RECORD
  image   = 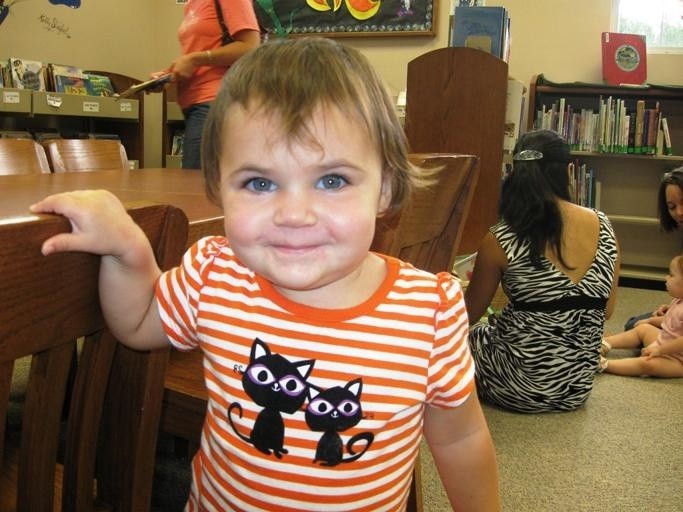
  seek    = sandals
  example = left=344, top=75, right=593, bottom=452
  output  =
left=597, top=339, right=612, bottom=374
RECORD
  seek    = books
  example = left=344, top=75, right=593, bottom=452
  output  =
left=535, top=95, right=673, bottom=156
left=601, top=31, right=647, bottom=87
left=113, top=72, right=175, bottom=103
left=0, top=55, right=115, bottom=96
left=447, top=5, right=510, bottom=64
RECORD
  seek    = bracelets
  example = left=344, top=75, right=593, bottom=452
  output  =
left=205, top=49, right=214, bottom=65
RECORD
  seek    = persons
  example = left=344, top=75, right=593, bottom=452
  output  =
left=30, top=34, right=506, bottom=512
left=146, top=1, right=264, bottom=169
left=657, top=166, right=683, bottom=232
left=463, top=130, right=623, bottom=416
left=597, top=253, right=683, bottom=380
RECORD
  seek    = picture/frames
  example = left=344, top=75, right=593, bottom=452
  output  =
left=252, top=0, right=440, bottom=39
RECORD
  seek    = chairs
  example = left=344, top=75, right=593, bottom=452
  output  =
left=0, top=135, right=482, bottom=512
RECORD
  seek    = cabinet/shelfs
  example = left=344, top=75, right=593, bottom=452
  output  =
left=1, top=69, right=185, bottom=170
left=404, top=47, right=526, bottom=254
left=520, top=75, right=683, bottom=283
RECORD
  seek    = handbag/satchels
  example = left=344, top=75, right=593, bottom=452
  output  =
left=223, top=32, right=273, bottom=69
